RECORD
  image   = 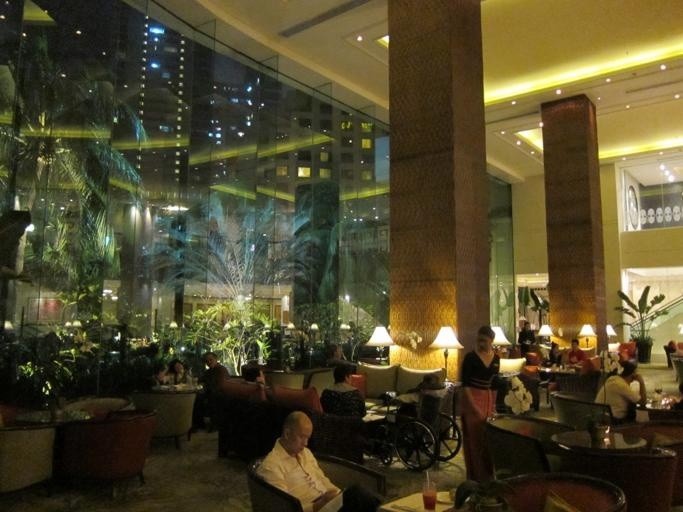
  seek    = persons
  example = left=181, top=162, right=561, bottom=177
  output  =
left=241, top=362, right=266, bottom=385
left=594, top=360, right=647, bottom=424
left=518, top=321, right=536, bottom=356
left=568, top=339, right=588, bottom=365
left=256, top=410, right=389, bottom=511
left=538, top=341, right=563, bottom=385
left=157, top=358, right=193, bottom=384
left=461, top=325, right=500, bottom=478
left=396, top=374, right=446, bottom=419
left=319, top=364, right=367, bottom=416
left=199, top=352, right=229, bottom=389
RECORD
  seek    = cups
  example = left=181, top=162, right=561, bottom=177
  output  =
left=655, top=383, right=663, bottom=394
left=422, top=481, right=438, bottom=510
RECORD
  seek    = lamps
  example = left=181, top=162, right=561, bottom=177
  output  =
left=432, top=326, right=464, bottom=369
left=607, top=325, right=617, bottom=342
left=490, top=324, right=511, bottom=352
left=366, top=326, right=393, bottom=364
left=539, top=324, right=552, bottom=344
left=579, top=323, right=596, bottom=347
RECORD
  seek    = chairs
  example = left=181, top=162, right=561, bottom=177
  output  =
left=0, top=340, right=683, bottom=512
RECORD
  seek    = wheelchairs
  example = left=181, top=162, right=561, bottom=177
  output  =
left=373, top=384, right=462, bottom=471
